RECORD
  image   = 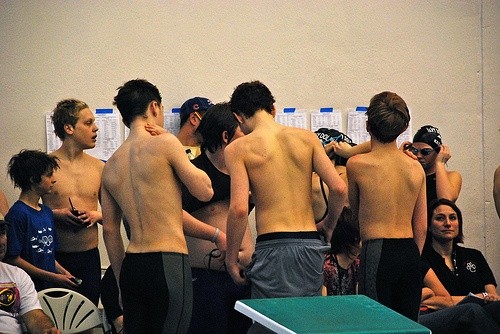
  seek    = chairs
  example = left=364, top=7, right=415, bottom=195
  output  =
left=37, top=287, right=104, bottom=334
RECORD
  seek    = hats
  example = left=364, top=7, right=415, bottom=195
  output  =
left=413, top=126, right=442, bottom=153
left=0, top=214, right=10, bottom=225
left=180, top=98, right=215, bottom=118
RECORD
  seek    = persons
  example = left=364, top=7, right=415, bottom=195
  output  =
left=312, top=128, right=372, bottom=296
left=400, top=125, right=500, bottom=334
left=346, top=91, right=427, bottom=322
left=223, top=81, right=347, bottom=334
left=100, top=215, right=131, bottom=334
left=0, top=190, right=62, bottom=334
left=182, top=103, right=256, bottom=334
left=176, top=97, right=214, bottom=160
left=101, top=80, right=215, bottom=334
left=40, top=99, right=105, bottom=334
left=493, top=166, right=500, bottom=220
left=1, top=149, right=78, bottom=328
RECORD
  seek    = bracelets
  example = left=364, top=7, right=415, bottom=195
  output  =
left=482, top=291, right=488, bottom=298
left=211, top=228, right=218, bottom=243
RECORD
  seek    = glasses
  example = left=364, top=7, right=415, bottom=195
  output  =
left=203, top=249, right=221, bottom=265
left=410, top=147, right=433, bottom=156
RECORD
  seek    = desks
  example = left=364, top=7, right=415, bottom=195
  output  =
left=234, top=295, right=432, bottom=334
